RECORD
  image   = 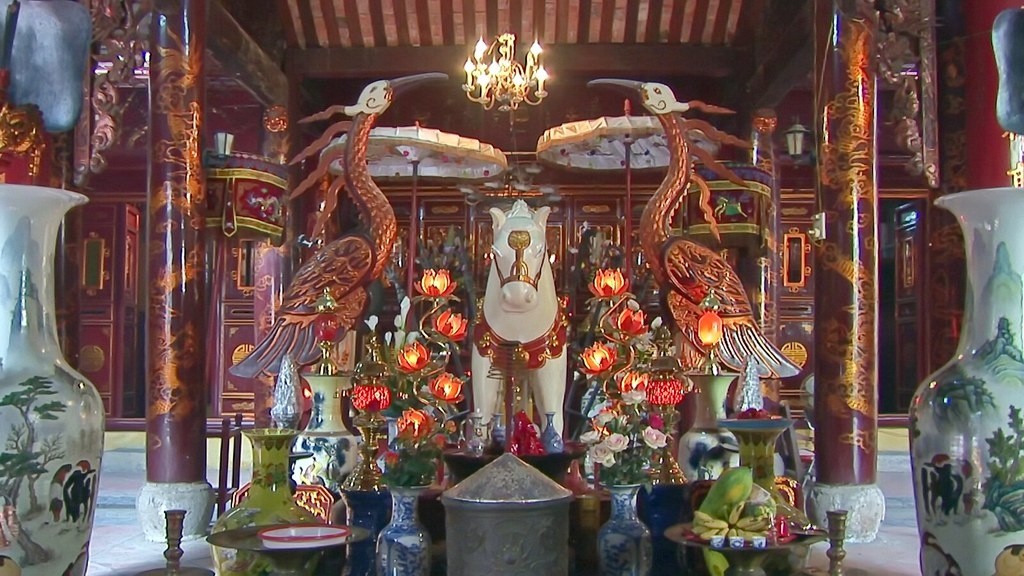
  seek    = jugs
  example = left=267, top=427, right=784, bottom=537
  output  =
left=457, top=408, right=491, bottom=458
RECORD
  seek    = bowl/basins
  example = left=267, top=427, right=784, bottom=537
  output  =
left=257, top=524, right=353, bottom=547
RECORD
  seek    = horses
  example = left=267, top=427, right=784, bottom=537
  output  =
left=471, top=200, right=567, bottom=439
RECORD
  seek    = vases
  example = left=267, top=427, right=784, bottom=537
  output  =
left=210, top=374, right=801, bottom=576
left=910, top=185, right=1024, bottom=576
left=0, top=183, right=106, bottom=576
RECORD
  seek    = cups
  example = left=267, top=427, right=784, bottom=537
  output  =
left=710, top=536, right=724, bottom=548
left=729, top=536, right=744, bottom=549
left=752, top=536, right=766, bottom=548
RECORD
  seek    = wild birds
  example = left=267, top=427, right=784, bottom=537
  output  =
left=589, top=78, right=803, bottom=379
left=227, top=73, right=449, bottom=379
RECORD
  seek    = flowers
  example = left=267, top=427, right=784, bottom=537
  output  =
left=583, top=267, right=651, bottom=401
left=397, top=265, right=469, bottom=434
left=364, top=294, right=424, bottom=413
left=630, top=298, right=675, bottom=359
left=579, top=384, right=670, bottom=490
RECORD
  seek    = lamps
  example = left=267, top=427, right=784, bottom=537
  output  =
left=203, top=129, right=235, bottom=166
left=780, top=113, right=813, bottom=169
left=462, top=29, right=549, bottom=114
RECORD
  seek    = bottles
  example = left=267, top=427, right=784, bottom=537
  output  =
left=492, top=414, right=507, bottom=453
left=909, top=188, right=1024, bottom=576
left=540, top=412, right=564, bottom=454
